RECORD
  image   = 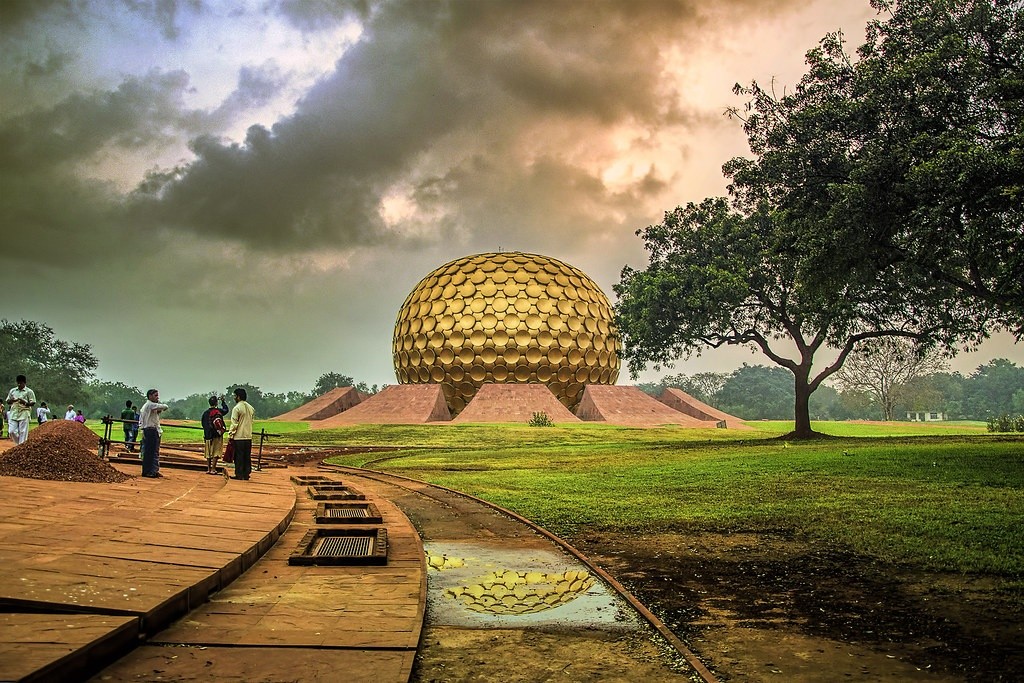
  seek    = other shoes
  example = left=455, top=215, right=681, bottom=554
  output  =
left=157, top=473, right=163, bottom=477
left=231, top=476, right=237, bottom=479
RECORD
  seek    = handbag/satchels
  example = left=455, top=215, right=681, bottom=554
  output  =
left=223, top=438, right=234, bottom=464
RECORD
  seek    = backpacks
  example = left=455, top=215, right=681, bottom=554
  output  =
left=209, top=407, right=226, bottom=436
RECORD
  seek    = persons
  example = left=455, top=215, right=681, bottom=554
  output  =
left=76, top=410, right=86, bottom=423
left=6, top=406, right=11, bottom=438
left=0, top=398, right=5, bottom=437
left=64, top=405, right=77, bottom=421
left=130, top=406, right=140, bottom=453
left=202, top=395, right=229, bottom=475
left=139, top=389, right=168, bottom=477
left=6, top=375, right=37, bottom=445
left=121, top=400, right=135, bottom=454
left=36, top=402, right=50, bottom=425
left=227, top=388, right=256, bottom=480
